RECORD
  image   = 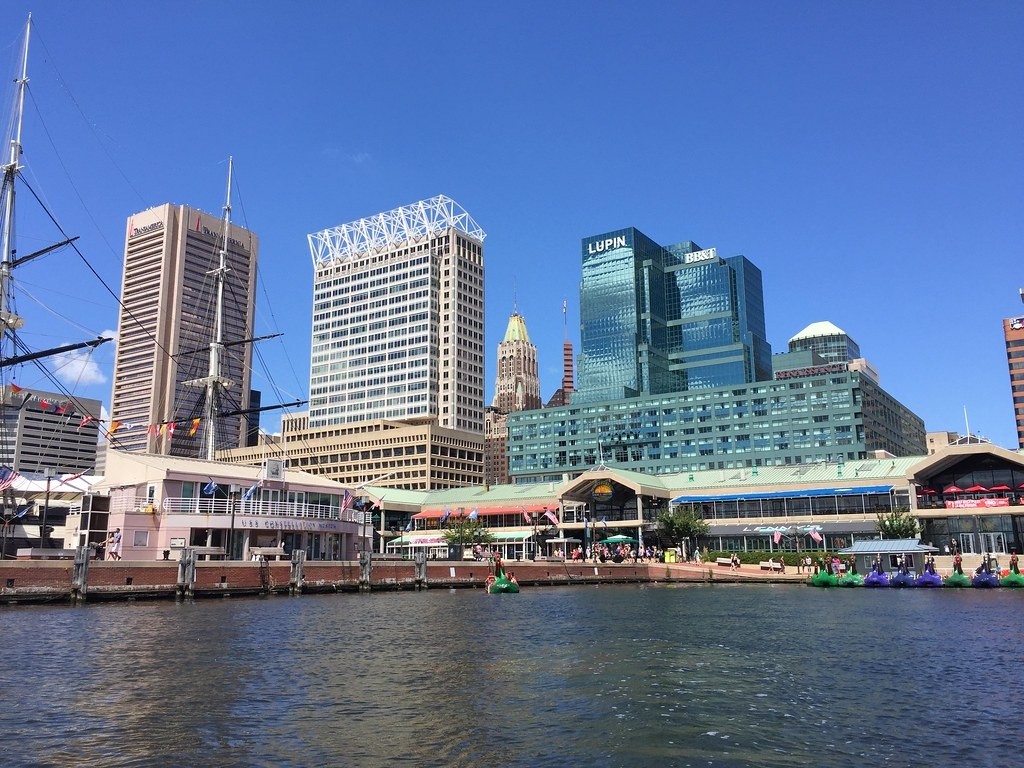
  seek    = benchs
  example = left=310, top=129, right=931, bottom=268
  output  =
left=187, top=546, right=230, bottom=561
left=474, top=552, right=496, bottom=562
left=760, top=562, right=781, bottom=571
left=547, top=557, right=565, bottom=563
left=357, top=553, right=403, bottom=561
left=715, top=557, right=741, bottom=568
left=249, top=547, right=289, bottom=561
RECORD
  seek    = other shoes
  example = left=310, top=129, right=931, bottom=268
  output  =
left=478, top=560, right=481, bottom=561
left=118, top=556, right=121, bottom=561
left=783, top=573, right=786, bottom=574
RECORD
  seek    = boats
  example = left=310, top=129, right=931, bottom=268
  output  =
left=485, top=552, right=520, bottom=594
left=811, top=557, right=838, bottom=587
left=945, top=553, right=973, bottom=588
left=839, top=555, right=864, bottom=587
left=918, top=552, right=943, bottom=587
left=865, top=553, right=890, bottom=587
left=974, top=554, right=1001, bottom=588
left=1000, top=552, right=1024, bottom=587
left=889, top=553, right=917, bottom=587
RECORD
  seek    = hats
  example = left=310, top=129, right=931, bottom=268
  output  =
left=579, top=545, right=582, bottom=548
left=488, top=573, right=495, bottom=578
left=587, top=546, right=589, bottom=549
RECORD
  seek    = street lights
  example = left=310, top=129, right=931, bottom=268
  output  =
left=458, top=507, right=465, bottom=545
left=41, top=467, right=57, bottom=548
left=532, top=510, right=538, bottom=556
left=399, top=525, right=405, bottom=548
left=591, top=516, right=597, bottom=563
left=361, top=496, right=369, bottom=551
left=227, top=484, right=240, bottom=560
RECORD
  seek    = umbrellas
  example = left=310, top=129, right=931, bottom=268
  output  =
left=917, top=483, right=1024, bottom=509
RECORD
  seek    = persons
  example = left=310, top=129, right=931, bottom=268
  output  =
left=798, top=553, right=841, bottom=576
left=100, top=528, right=122, bottom=561
left=475, top=543, right=492, bottom=562
left=730, top=553, right=741, bottom=572
left=554, top=542, right=708, bottom=564
left=767, top=556, right=785, bottom=574
left=266, top=537, right=286, bottom=561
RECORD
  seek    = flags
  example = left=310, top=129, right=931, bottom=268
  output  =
left=440, top=510, right=452, bottom=524
left=16, top=505, right=34, bottom=519
left=809, top=528, right=822, bottom=545
left=0, top=463, right=21, bottom=491
left=204, top=480, right=221, bottom=497
left=371, top=495, right=384, bottom=510
left=544, top=510, right=561, bottom=525
left=9, top=377, right=204, bottom=443
left=521, top=506, right=532, bottom=523
left=774, top=531, right=781, bottom=544
left=340, top=490, right=353, bottom=514
left=467, top=507, right=478, bottom=520
left=240, top=480, right=266, bottom=500
left=60, top=467, right=93, bottom=486
